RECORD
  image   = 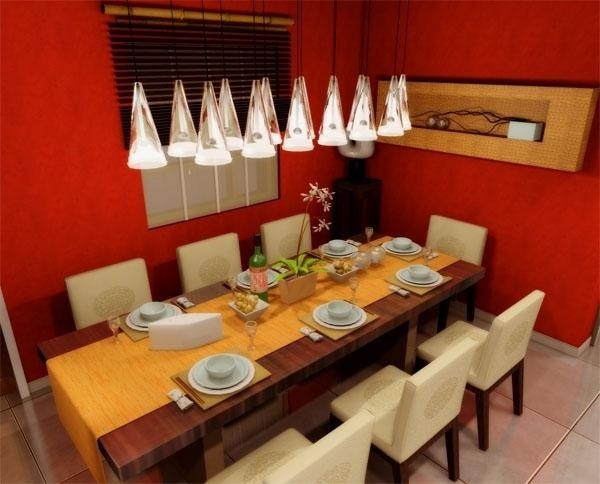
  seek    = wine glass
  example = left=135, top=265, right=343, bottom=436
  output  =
left=349, top=275, right=362, bottom=303
left=365, top=228, right=374, bottom=245
left=244, top=319, right=258, bottom=352
left=108, top=314, right=123, bottom=348
left=422, top=243, right=434, bottom=267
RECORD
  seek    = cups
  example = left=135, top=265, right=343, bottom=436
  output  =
left=355, top=248, right=383, bottom=274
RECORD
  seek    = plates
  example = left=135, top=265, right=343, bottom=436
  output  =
left=189, top=353, right=256, bottom=396
left=319, top=243, right=358, bottom=257
left=396, top=263, right=444, bottom=288
left=125, top=303, right=182, bottom=332
left=314, top=300, right=366, bottom=330
left=379, top=240, right=423, bottom=256
left=235, top=269, right=281, bottom=289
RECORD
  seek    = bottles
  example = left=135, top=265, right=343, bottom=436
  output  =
left=249, top=233, right=269, bottom=303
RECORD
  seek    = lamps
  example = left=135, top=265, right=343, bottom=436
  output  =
left=125, top=74, right=169, bottom=172
left=398, top=73, right=411, bottom=132
left=262, top=76, right=283, bottom=146
left=241, top=75, right=277, bottom=159
left=193, top=80, right=232, bottom=166
left=347, top=74, right=378, bottom=142
left=377, top=76, right=406, bottom=137
left=164, top=78, right=199, bottom=158
left=216, top=75, right=243, bottom=152
left=319, top=72, right=350, bottom=145
left=282, top=78, right=314, bottom=152
left=299, top=75, right=316, bottom=139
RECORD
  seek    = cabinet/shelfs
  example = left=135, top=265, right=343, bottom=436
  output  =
left=376, top=77, right=597, bottom=175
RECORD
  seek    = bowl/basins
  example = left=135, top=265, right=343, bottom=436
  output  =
left=328, top=239, right=347, bottom=251
left=227, top=296, right=271, bottom=322
left=323, top=262, right=360, bottom=283
left=392, top=236, right=412, bottom=248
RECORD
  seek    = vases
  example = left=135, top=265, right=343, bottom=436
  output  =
left=278, top=270, right=319, bottom=305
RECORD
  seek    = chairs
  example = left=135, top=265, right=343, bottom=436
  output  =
left=261, top=213, right=312, bottom=263
left=416, top=288, right=546, bottom=451
left=203, top=408, right=372, bottom=484
left=65, top=257, right=151, bottom=331
left=175, top=231, right=242, bottom=293
left=331, top=336, right=477, bottom=483
left=425, top=215, right=488, bottom=332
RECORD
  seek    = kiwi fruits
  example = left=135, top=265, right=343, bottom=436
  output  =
left=334, top=261, right=353, bottom=275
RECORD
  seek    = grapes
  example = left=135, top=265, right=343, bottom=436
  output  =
left=230, top=289, right=260, bottom=314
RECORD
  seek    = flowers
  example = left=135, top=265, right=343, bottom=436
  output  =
left=272, top=181, right=336, bottom=282
